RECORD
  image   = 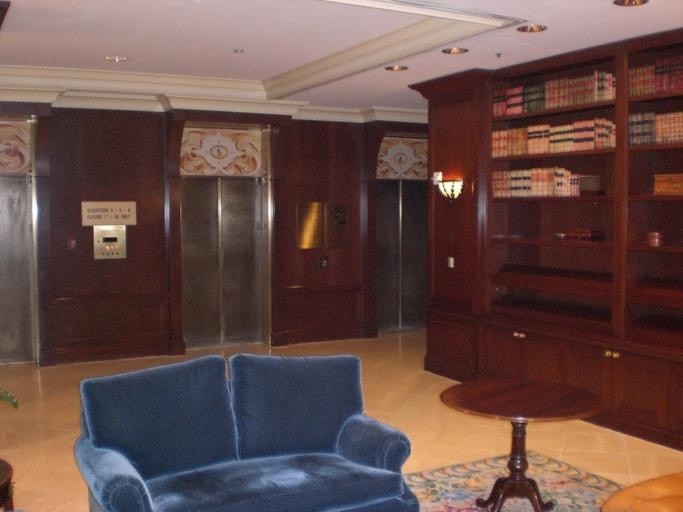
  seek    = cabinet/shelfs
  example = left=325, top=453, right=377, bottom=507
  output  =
left=622, top=43, right=682, bottom=355
left=477, top=320, right=560, bottom=389
left=555, top=336, right=682, bottom=436
left=490, top=52, right=623, bottom=357
left=428, top=87, right=491, bottom=318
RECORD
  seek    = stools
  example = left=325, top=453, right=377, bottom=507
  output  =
left=1, top=459, right=16, bottom=510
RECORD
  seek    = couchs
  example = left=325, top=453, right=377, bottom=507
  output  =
left=73, top=352, right=419, bottom=512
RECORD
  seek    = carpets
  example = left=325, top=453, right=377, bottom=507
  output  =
left=403, top=450, right=623, bottom=512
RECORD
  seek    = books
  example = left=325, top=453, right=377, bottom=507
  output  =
left=490, top=56, right=683, bottom=196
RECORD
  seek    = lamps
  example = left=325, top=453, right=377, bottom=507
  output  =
left=431, top=177, right=465, bottom=204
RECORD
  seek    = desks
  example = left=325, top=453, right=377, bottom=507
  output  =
left=439, top=379, right=612, bottom=511
